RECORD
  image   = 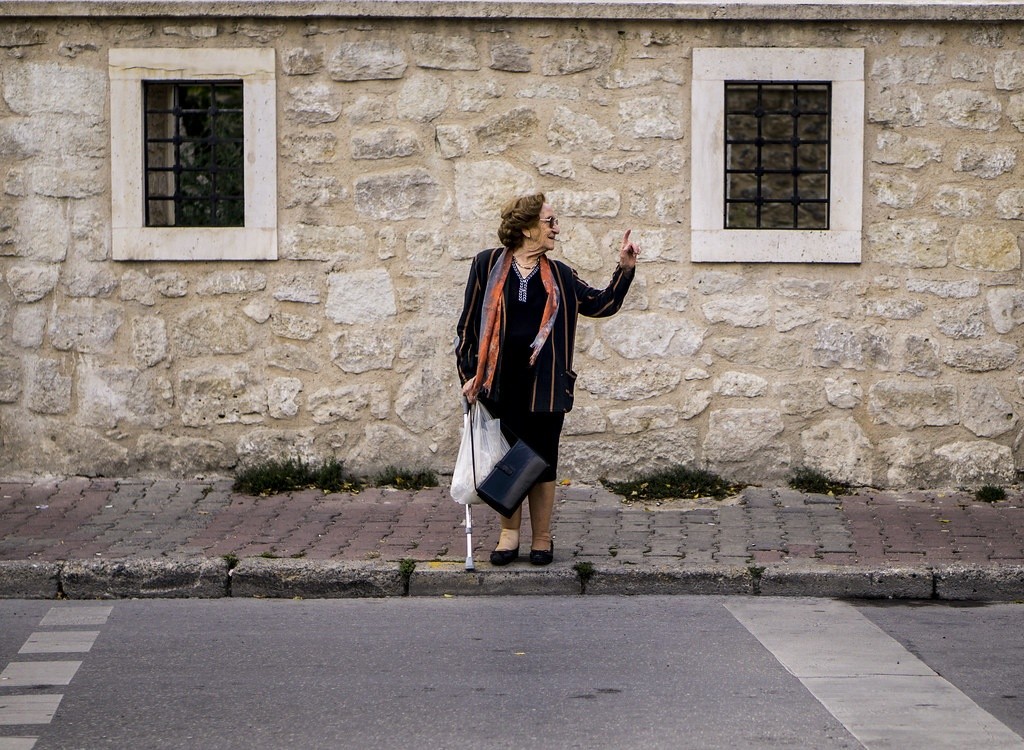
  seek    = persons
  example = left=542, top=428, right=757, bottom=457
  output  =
left=455, top=192, right=641, bottom=567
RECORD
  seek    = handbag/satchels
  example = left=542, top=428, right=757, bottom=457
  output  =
left=450, top=399, right=511, bottom=505
left=468, top=402, right=548, bottom=519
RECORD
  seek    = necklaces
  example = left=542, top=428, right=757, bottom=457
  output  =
left=512, top=254, right=540, bottom=269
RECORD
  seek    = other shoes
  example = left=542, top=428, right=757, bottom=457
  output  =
left=530, top=539, right=554, bottom=565
left=490, top=539, right=520, bottom=565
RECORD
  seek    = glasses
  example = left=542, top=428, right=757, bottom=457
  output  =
left=538, top=216, right=560, bottom=228
